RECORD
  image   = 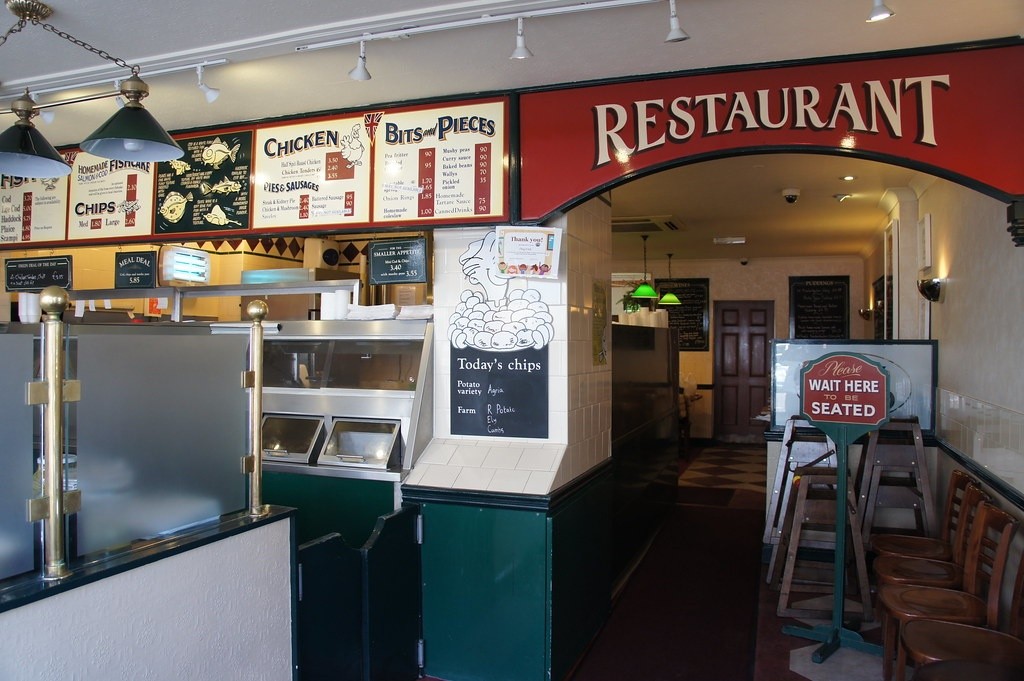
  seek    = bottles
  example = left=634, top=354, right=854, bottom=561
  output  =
left=683, top=372, right=698, bottom=396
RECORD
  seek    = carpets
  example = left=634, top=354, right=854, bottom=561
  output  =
left=562, top=504, right=764, bottom=681
left=675, top=484, right=766, bottom=507
left=679, top=446, right=705, bottom=476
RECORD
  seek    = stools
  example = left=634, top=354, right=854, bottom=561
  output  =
left=767, top=417, right=936, bottom=622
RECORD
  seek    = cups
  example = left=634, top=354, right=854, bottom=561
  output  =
left=619, top=306, right=668, bottom=327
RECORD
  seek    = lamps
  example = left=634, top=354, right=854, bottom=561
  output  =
left=658, top=254, right=681, bottom=304
left=508, top=18, right=533, bottom=59
left=193, top=66, right=221, bottom=102
left=113, top=80, right=125, bottom=108
left=32, top=16, right=185, bottom=162
left=346, top=40, right=371, bottom=81
left=0, top=87, right=72, bottom=178
left=31, top=92, right=54, bottom=124
left=664, top=0, right=689, bottom=43
left=630, top=234, right=659, bottom=299
left=865, top=0, right=895, bottom=22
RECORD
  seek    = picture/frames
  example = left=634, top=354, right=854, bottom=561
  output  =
left=5, top=255, right=73, bottom=293
left=883, top=219, right=899, bottom=341
left=368, top=237, right=428, bottom=285
left=115, top=251, right=157, bottom=288
left=789, top=275, right=850, bottom=339
left=916, top=214, right=931, bottom=271
left=654, top=277, right=710, bottom=352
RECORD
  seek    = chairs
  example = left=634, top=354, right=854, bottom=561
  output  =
left=870, top=469, right=1024, bottom=681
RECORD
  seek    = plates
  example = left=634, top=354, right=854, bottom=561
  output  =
left=18, top=293, right=43, bottom=323
left=320, top=290, right=350, bottom=320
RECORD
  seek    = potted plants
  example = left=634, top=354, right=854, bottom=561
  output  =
left=616, top=280, right=652, bottom=310
